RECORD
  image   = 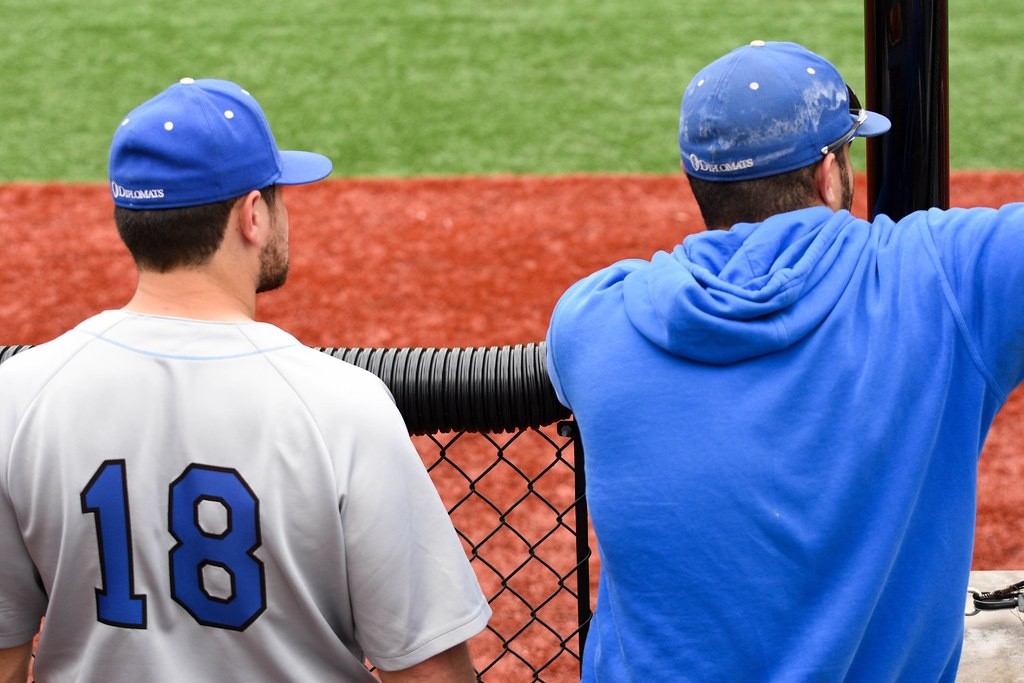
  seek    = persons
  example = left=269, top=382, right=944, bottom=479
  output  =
left=545, top=37, right=1023, bottom=682
left=0, top=74, right=497, bottom=682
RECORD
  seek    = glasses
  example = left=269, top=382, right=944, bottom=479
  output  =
left=820, top=81, right=867, bottom=156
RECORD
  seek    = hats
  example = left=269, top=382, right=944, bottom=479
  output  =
left=110, top=78, right=333, bottom=209
left=680, top=39, right=891, bottom=182
